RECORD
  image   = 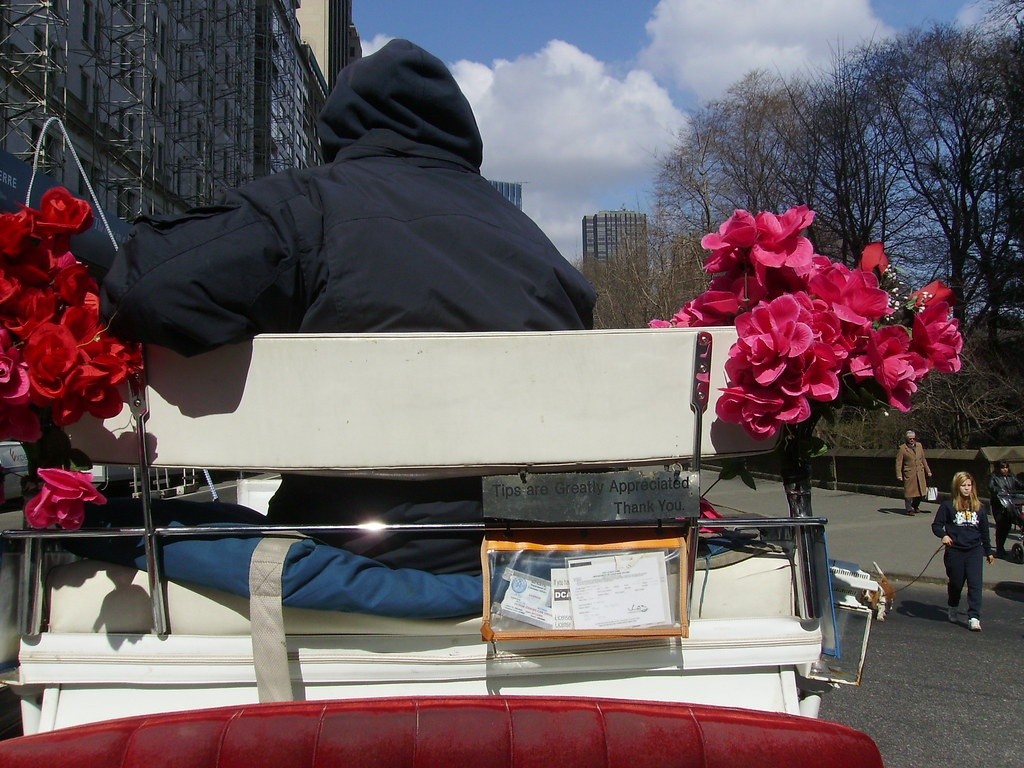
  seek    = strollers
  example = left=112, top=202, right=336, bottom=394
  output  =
left=997, top=488, right=1024, bottom=565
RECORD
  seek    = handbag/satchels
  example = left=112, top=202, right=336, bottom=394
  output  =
left=926, top=475, right=938, bottom=500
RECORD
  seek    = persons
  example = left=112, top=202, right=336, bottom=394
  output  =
left=931, top=471, right=994, bottom=631
left=112, top=40, right=592, bottom=575
left=896, top=431, right=933, bottom=515
left=987, top=459, right=1024, bottom=559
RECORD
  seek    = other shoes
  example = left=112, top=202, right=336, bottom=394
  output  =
left=907, top=509, right=915, bottom=516
left=911, top=503, right=920, bottom=513
left=996, top=548, right=1008, bottom=556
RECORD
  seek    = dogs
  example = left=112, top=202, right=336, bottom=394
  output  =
left=860, top=561, right=892, bottom=621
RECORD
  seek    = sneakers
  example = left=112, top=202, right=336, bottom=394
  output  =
left=968, top=617, right=981, bottom=630
left=948, top=606, right=958, bottom=622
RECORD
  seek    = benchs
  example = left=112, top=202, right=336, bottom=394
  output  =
left=0, top=324, right=829, bottom=736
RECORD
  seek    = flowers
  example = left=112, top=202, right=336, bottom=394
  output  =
left=0, top=185, right=144, bottom=532
left=648, top=204, right=962, bottom=533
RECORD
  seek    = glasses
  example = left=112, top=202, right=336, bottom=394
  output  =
left=907, top=438, right=915, bottom=440
